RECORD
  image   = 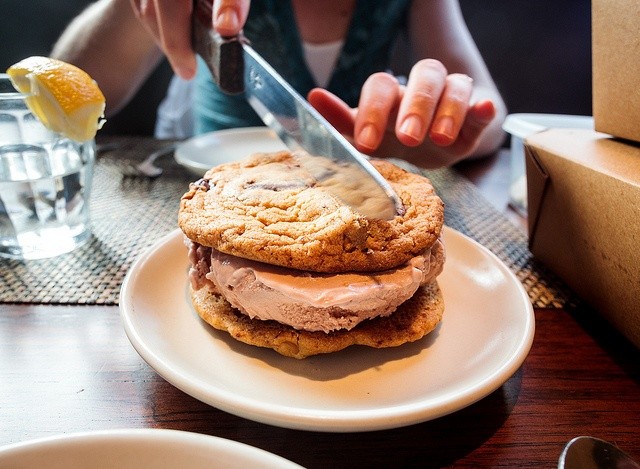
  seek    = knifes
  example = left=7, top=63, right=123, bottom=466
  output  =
left=182, top=1, right=406, bottom=224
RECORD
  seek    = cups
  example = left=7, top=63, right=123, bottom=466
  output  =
left=0, top=70, right=97, bottom=260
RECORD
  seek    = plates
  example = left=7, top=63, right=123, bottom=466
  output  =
left=0, top=429, right=304, bottom=469
left=172, top=127, right=293, bottom=178
left=117, top=226, right=536, bottom=432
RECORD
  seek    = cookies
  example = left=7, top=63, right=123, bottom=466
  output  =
left=179, top=155, right=445, bottom=275
left=190, top=279, right=445, bottom=361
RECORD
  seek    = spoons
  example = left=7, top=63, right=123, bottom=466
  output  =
left=555, top=430, right=636, bottom=467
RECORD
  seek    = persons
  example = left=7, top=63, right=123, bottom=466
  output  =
left=46, top=0, right=507, bottom=168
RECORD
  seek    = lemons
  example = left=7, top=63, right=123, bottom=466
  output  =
left=7, top=55, right=107, bottom=144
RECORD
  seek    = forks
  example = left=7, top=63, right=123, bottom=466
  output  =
left=111, top=140, right=176, bottom=180
